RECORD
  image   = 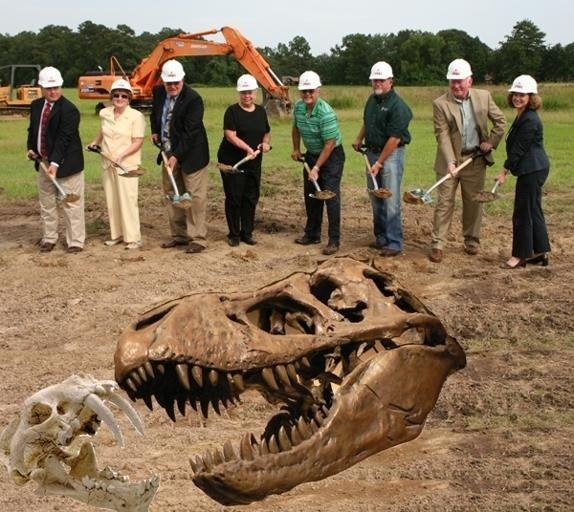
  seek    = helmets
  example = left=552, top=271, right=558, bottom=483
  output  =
left=236, top=73, right=259, bottom=92
left=369, top=60, right=394, bottom=80
left=507, top=74, right=538, bottom=94
left=111, top=78, right=133, bottom=94
left=297, top=70, right=322, bottom=91
left=446, top=58, right=473, bottom=80
left=37, top=66, right=64, bottom=89
left=160, top=58, right=185, bottom=83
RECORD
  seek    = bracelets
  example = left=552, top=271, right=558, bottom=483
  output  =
left=313, top=166, right=321, bottom=172
left=375, top=161, right=383, bottom=168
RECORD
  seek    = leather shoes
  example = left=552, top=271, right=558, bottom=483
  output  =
left=368, top=242, right=387, bottom=249
left=228, top=236, right=240, bottom=247
left=67, top=246, right=82, bottom=252
left=295, top=235, right=321, bottom=245
left=39, top=243, right=53, bottom=252
left=124, top=241, right=143, bottom=249
left=464, top=244, right=478, bottom=255
left=430, top=248, right=442, bottom=263
left=243, top=235, right=257, bottom=245
left=322, top=241, right=339, bottom=255
left=103, top=238, right=120, bottom=246
left=379, top=249, right=402, bottom=257
left=186, top=243, right=205, bottom=253
left=161, top=240, right=188, bottom=248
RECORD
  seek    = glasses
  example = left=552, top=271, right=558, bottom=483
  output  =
left=112, top=94, right=128, bottom=99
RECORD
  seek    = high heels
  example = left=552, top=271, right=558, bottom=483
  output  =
left=498, top=256, right=529, bottom=269
left=527, top=253, right=549, bottom=266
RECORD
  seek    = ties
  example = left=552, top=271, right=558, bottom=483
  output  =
left=163, top=97, right=175, bottom=152
left=40, top=103, right=52, bottom=156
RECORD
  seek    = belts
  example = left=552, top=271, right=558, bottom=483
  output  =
left=460, top=147, right=479, bottom=156
left=369, top=146, right=382, bottom=154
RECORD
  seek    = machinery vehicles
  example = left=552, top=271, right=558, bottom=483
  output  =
left=0, top=63, right=44, bottom=115
left=78, top=26, right=294, bottom=123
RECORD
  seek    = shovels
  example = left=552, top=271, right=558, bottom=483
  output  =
left=298, top=153, right=336, bottom=199
left=28, top=153, right=81, bottom=202
left=153, top=137, right=194, bottom=209
left=88, top=144, right=148, bottom=178
left=358, top=144, right=393, bottom=199
left=403, top=145, right=492, bottom=205
left=471, top=168, right=510, bottom=203
left=215, top=146, right=273, bottom=174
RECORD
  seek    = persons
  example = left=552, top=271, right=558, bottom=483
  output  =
left=353, top=61, right=413, bottom=258
left=216, top=74, right=272, bottom=247
left=149, top=59, right=210, bottom=253
left=85, top=79, right=145, bottom=250
left=429, top=59, right=506, bottom=263
left=291, top=70, right=345, bottom=255
left=495, top=74, right=551, bottom=271
left=26, top=66, right=86, bottom=253
